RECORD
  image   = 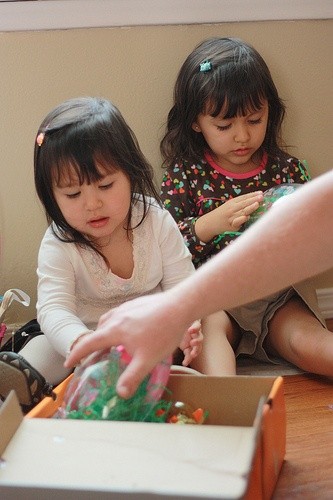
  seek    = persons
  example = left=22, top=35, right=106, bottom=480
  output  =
left=0, top=97, right=206, bottom=407
left=63, top=169, right=333, bottom=398
left=159, top=37, right=333, bottom=379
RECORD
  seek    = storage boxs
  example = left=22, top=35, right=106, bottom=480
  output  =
left=0, top=360, right=288, bottom=500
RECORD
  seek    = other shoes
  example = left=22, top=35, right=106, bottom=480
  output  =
left=0, top=352, right=54, bottom=415
